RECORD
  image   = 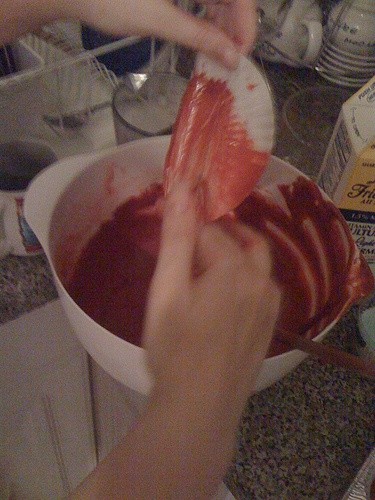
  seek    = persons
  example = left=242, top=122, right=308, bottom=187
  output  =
left=0, top=0, right=285, bottom=500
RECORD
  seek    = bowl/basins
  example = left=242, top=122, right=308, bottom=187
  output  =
left=328, top=1, right=375, bottom=53
left=25, top=131, right=373, bottom=402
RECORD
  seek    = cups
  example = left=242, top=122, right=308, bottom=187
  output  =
left=275, top=83, right=353, bottom=181
left=1, top=138, right=65, bottom=260
left=113, top=67, right=194, bottom=141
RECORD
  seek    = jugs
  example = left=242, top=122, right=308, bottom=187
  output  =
left=251, top=0, right=324, bottom=68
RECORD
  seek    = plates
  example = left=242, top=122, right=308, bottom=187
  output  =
left=315, top=23, right=375, bottom=89
